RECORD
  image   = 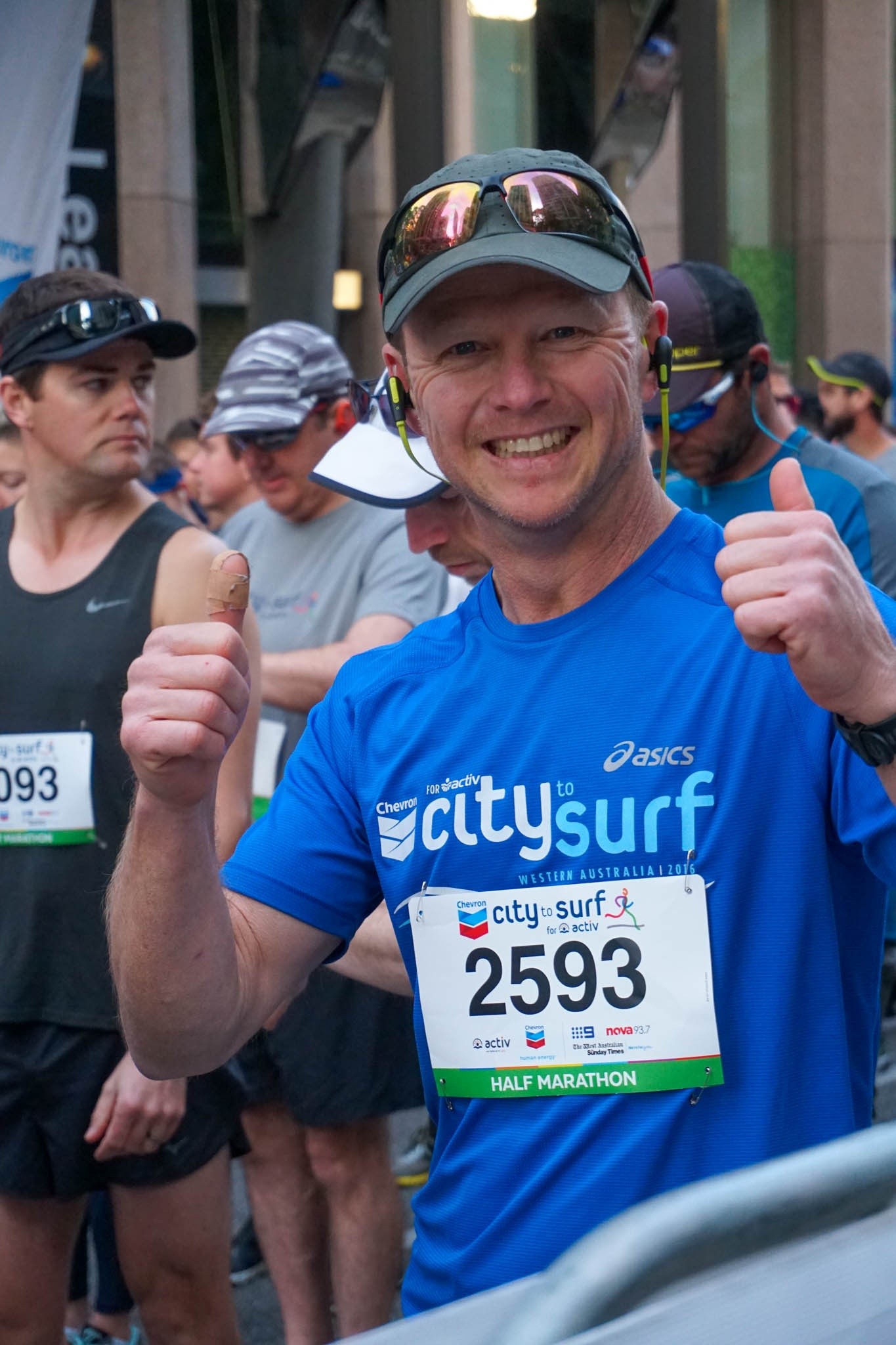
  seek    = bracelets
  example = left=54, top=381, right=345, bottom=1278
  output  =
left=834, top=712, right=896, bottom=767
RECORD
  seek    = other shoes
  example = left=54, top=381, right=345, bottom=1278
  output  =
left=63, top=1323, right=141, bottom=1345
left=228, top=1216, right=268, bottom=1284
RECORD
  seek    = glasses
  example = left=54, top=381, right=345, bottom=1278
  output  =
left=228, top=400, right=334, bottom=457
left=642, top=358, right=743, bottom=436
left=344, top=377, right=397, bottom=428
left=0, top=297, right=162, bottom=369
left=377, top=166, right=655, bottom=309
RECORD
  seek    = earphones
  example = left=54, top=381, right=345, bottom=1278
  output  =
left=388, top=375, right=415, bottom=427
left=642, top=335, right=674, bottom=393
left=748, top=359, right=769, bottom=387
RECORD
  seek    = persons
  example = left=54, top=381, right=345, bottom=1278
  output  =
left=104, top=150, right=896, bottom=1345
left=1, top=255, right=896, bottom=1345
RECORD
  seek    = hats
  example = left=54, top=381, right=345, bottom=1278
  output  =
left=804, top=350, right=892, bottom=408
left=306, top=366, right=450, bottom=510
left=198, top=320, right=356, bottom=441
left=377, top=148, right=654, bottom=338
left=639, top=258, right=769, bottom=416
left=0, top=288, right=198, bottom=377
